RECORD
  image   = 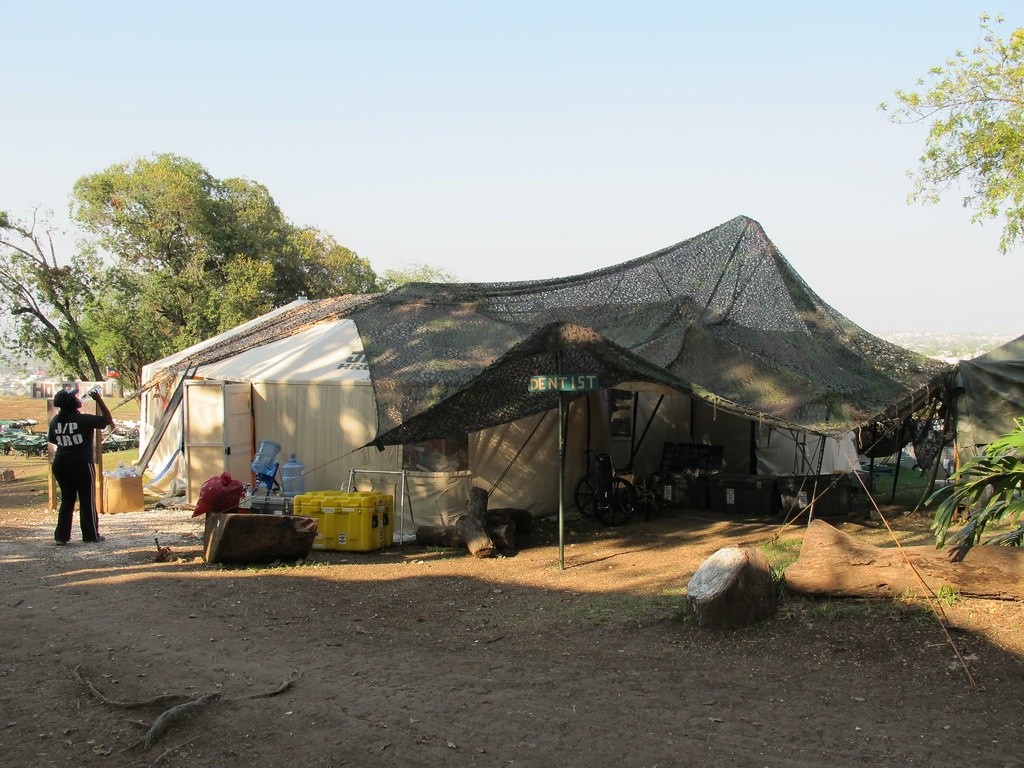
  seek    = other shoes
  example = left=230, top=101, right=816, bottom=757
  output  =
left=84, top=536, right=105, bottom=542
left=56, top=538, right=69, bottom=545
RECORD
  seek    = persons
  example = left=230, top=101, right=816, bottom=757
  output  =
left=48, top=389, right=112, bottom=545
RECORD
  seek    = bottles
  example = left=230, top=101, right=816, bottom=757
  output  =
left=80, top=384, right=102, bottom=402
left=341, top=483, right=347, bottom=490
left=248, top=437, right=281, bottom=478
left=103, top=458, right=145, bottom=478
left=281, top=452, right=304, bottom=496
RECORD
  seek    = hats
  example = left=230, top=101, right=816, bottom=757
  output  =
left=53, top=388, right=79, bottom=407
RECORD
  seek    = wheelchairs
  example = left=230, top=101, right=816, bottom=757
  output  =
left=573, top=448, right=665, bottom=527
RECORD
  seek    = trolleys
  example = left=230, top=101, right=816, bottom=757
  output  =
left=0, top=417, right=140, bottom=459
